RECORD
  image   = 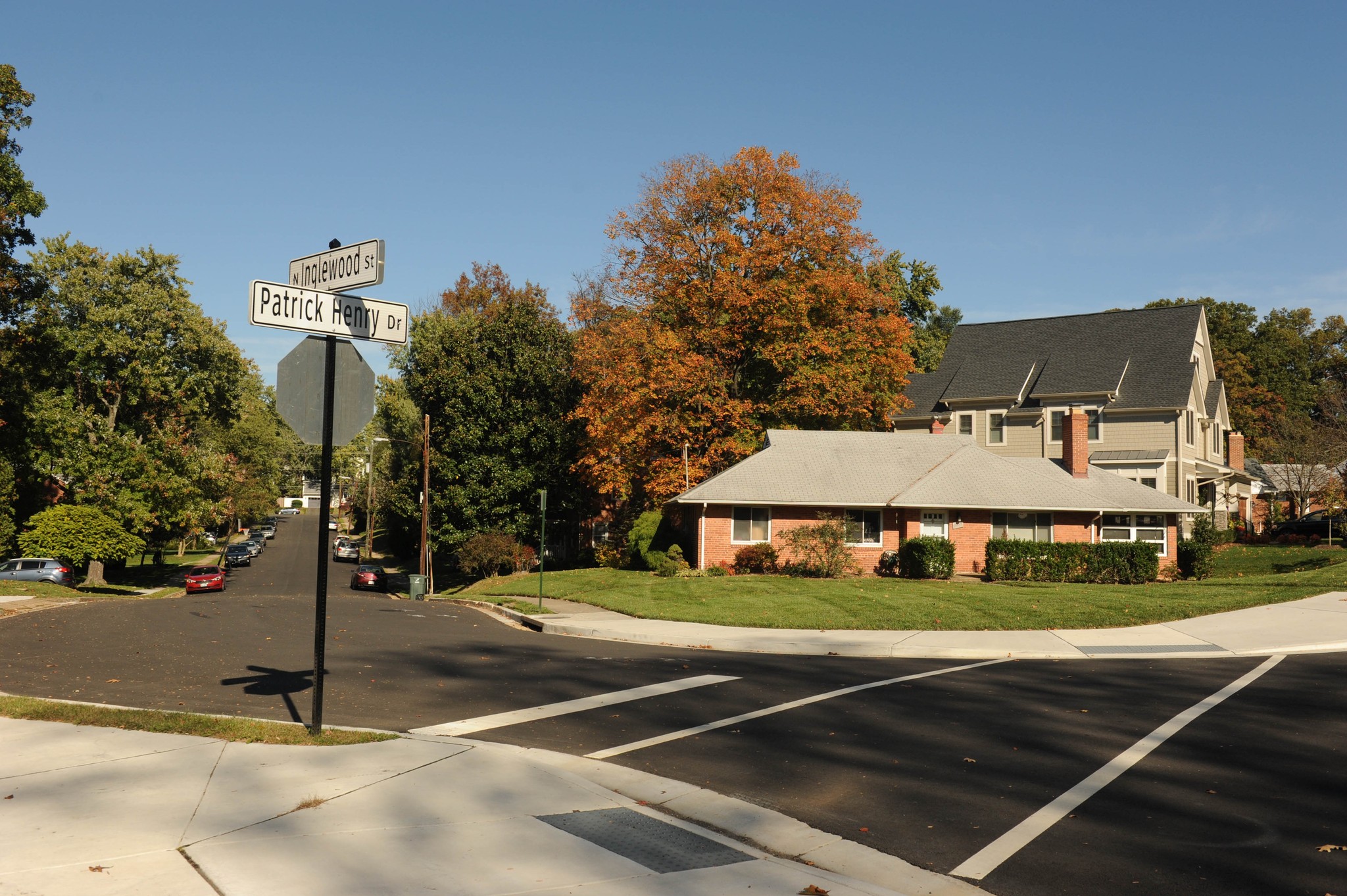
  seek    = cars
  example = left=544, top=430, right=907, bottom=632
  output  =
left=266, top=516, right=277, bottom=525
left=200, top=532, right=218, bottom=545
left=350, top=565, right=388, bottom=593
left=245, top=538, right=264, bottom=553
left=329, top=520, right=339, bottom=531
left=275, top=507, right=300, bottom=515
left=221, top=545, right=253, bottom=567
left=184, top=565, right=227, bottom=594
left=264, top=521, right=277, bottom=531
left=332, top=535, right=350, bottom=548
left=249, top=532, right=267, bottom=546
left=238, top=540, right=259, bottom=557
left=259, top=525, right=276, bottom=539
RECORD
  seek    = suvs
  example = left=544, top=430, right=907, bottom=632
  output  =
left=333, top=539, right=360, bottom=564
left=0, top=557, right=76, bottom=589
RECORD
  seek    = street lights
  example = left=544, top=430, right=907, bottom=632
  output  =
left=372, top=437, right=429, bottom=575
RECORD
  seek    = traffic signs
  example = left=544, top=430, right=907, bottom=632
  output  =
left=289, top=238, right=386, bottom=294
left=249, top=279, right=410, bottom=345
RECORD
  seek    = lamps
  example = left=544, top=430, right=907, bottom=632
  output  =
left=957, top=513, right=962, bottom=522
left=931, top=513, right=935, bottom=524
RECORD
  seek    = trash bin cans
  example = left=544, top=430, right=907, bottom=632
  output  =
left=408, top=574, right=428, bottom=601
